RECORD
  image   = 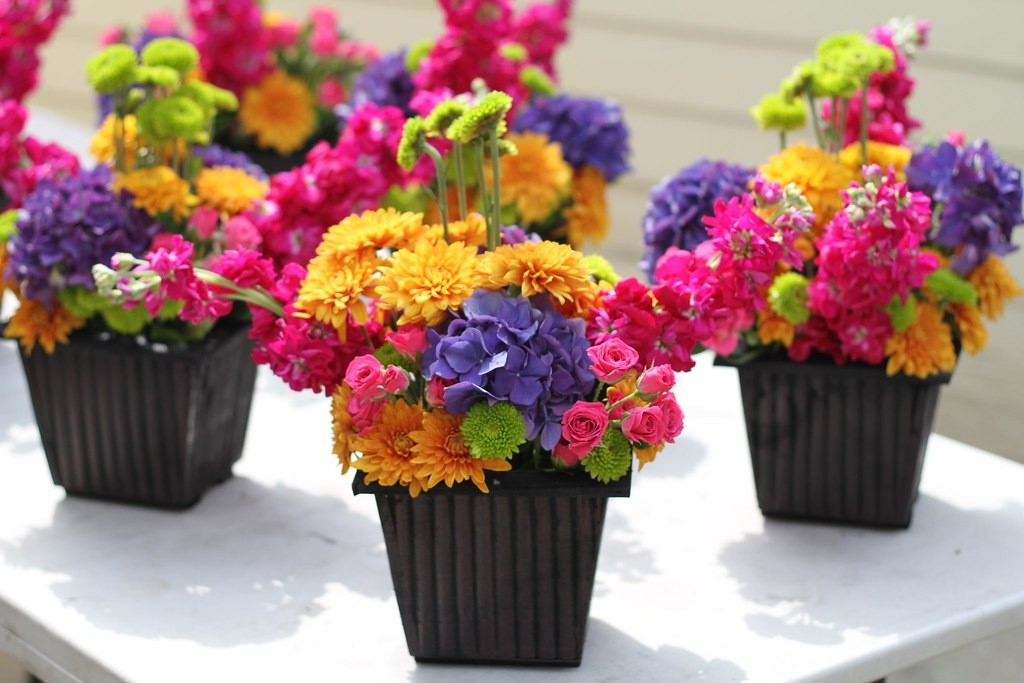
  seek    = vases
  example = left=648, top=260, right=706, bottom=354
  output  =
left=0, top=304, right=258, bottom=513
left=349, top=453, right=632, bottom=667
left=711, top=337, right=959, bottom=533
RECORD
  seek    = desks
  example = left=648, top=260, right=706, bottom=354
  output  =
left=0, top=157, right=1024, bottom=683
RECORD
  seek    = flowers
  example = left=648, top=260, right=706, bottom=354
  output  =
left=0, top=0, right=1022, bottom=495
